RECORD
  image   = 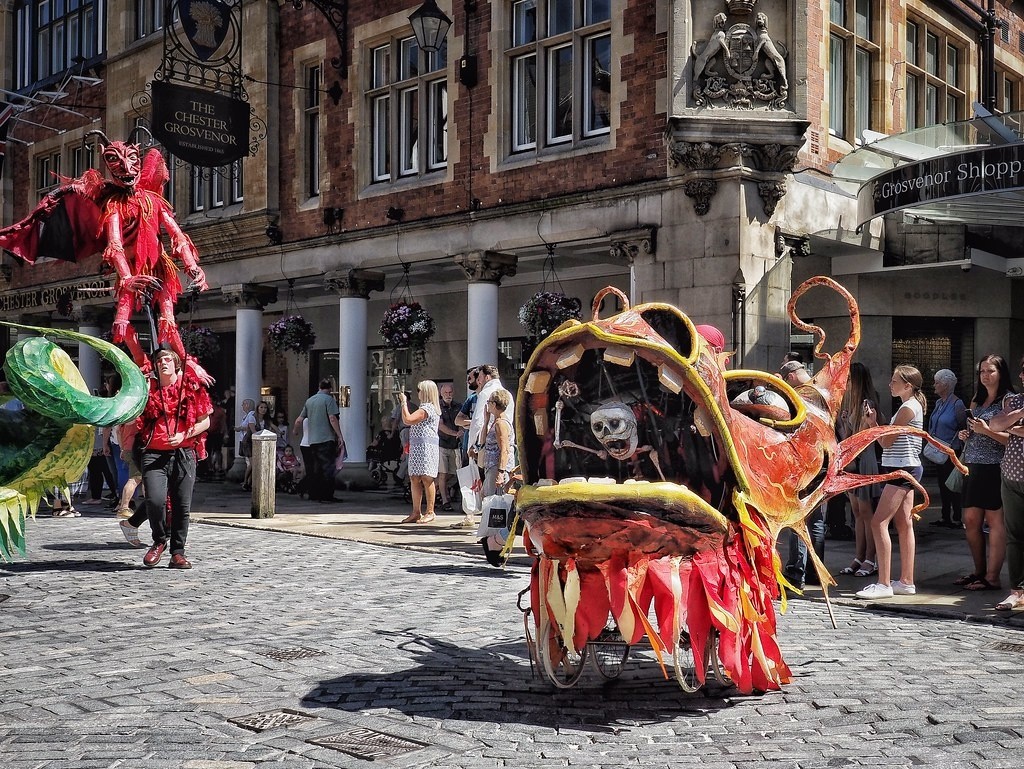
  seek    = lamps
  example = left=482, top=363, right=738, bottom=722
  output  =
left=406, top=0, right=480, bottom=87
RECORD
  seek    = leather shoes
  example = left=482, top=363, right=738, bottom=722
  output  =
left=143, top=541, right=167, bottom=566
left=169, top=554, right=192, bottom=569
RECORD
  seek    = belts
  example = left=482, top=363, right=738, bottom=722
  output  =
left=400, top=427, right=410, bottom=432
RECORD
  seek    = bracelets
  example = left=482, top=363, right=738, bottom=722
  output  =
left=120, top=449, right=127, bottom=454
left=498, top=468, right=506, bottom=473
left=401, top=402, right=407, bottom=407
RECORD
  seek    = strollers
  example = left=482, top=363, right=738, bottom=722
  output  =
left=404, top=481, right=451, bottom=505
left=277, top=430, right=304, bottom=494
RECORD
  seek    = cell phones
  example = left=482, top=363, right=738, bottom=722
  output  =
left=863, top=399, right=870, bottom=418
left=964, top=409, right=975, bottom=423
left=402, top=386, right=404, bottom=395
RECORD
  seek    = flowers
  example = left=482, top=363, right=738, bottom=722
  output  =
left=517, top=291, right=584, bottom=348
left=268, top=315, right=318, bottom=370
left=180, top=325, right=220, bottom=361
left=377, top=298, right=436, bottom=374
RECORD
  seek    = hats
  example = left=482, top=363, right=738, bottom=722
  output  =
left=779, top=361, right=805, bottom=380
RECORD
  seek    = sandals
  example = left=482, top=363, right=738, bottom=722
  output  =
left=995, top=592, right=1024, bottom=610
left=840, top=557, right=879, bottom=577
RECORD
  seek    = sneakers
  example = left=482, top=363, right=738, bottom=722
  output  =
left=890, top=580, right=916, bottom=595
left=856, top=583, right=893, bottom=599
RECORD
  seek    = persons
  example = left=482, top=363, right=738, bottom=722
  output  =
left=196, top=387, right=305, bottom=494
left=726, top=353, right=1024, bottom=610
left=292, top=377, right=348, bottom=502
left=52, top=373, right=171, bottom=547
left=368, top=365, right=514, bottom=567
left=143, top=348, right=213, bottom=569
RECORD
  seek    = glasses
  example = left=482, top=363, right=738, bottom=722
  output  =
left=156, top=358, right=173, bottom=363
left=468, top=377, right=475, bottom=381
left=417, top=389, right=421, bottom=393
left=1021, top=367, right=1024, bottom=374
left=487, top=400, right=497, bottom=405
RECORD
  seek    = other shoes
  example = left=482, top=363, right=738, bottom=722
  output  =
left=49, top=491, right=145, bottom=518
left=399, top=496, right=491, bottom=544
left=780, top=571, right=820, bottom=590
left=983, top=522, right=990, bottom=534
left=320, top=495, right=341, bottom=505
left=946, top=522, right=963, bottom=530
left=929, top=519, right=952, bottom=526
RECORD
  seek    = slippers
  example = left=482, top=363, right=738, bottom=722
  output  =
left=953, top=574, right=982, bottom=585
left=120, top=520, right=140, bottom=547
left=963, top=579, right=1002, bottom=590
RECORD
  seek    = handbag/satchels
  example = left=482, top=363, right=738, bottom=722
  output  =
left=476, top=486, right=514, bottom=537
left=239, top=441, right=252, bottom=456
left=944, top=453, right=965, bottom=493
left=923, top=435, right=952, bottom=464
left=456, top=456, right=483, bottom=514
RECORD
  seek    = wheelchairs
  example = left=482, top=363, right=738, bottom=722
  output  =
left=366, top=426, right=406, bottom=484
